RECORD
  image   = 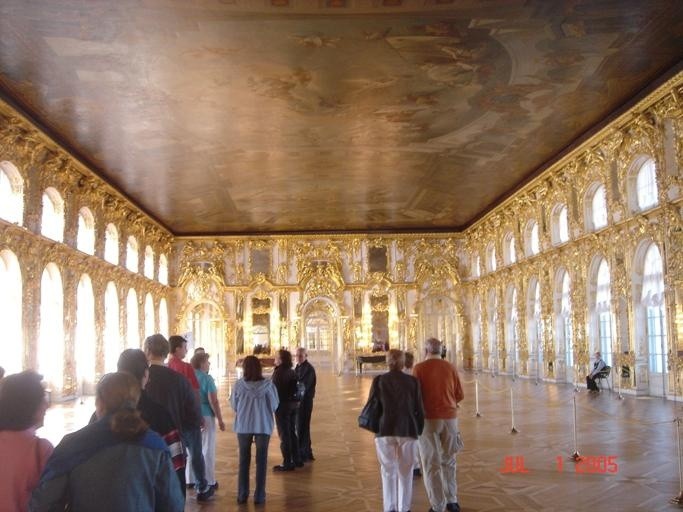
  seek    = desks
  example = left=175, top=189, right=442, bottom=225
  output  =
left=236, top=356, right=277, bottom=369
left=357, top=353, right=387, bottom=375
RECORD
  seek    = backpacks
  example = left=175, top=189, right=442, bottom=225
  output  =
left=289, top=378, right=306, bottom=402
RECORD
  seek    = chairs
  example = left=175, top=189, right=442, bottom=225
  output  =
left=595, top=365, right=612, bottom=392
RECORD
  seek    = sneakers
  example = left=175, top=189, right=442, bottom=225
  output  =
left=272, top=458, right=306, bottom=471
left=447, top=502, right=462, bottom=511
left=197, top=485, right=215, bottom=501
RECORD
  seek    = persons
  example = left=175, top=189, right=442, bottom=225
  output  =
left=358, top=349, right=425, bottom=512
left=229, top=355, right=281, bottom=505
left=401, top=351, right=421, bottom=477
left=295, top=348, right=316, bottom=462
left=586, top=352, right=606, bottom=393
left=411, top=338, right=464, bottom=512
left=270, top=349, right=305, bottom=473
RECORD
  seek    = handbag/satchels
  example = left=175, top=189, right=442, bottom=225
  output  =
left=357, top=392, right=384, bottom=433
